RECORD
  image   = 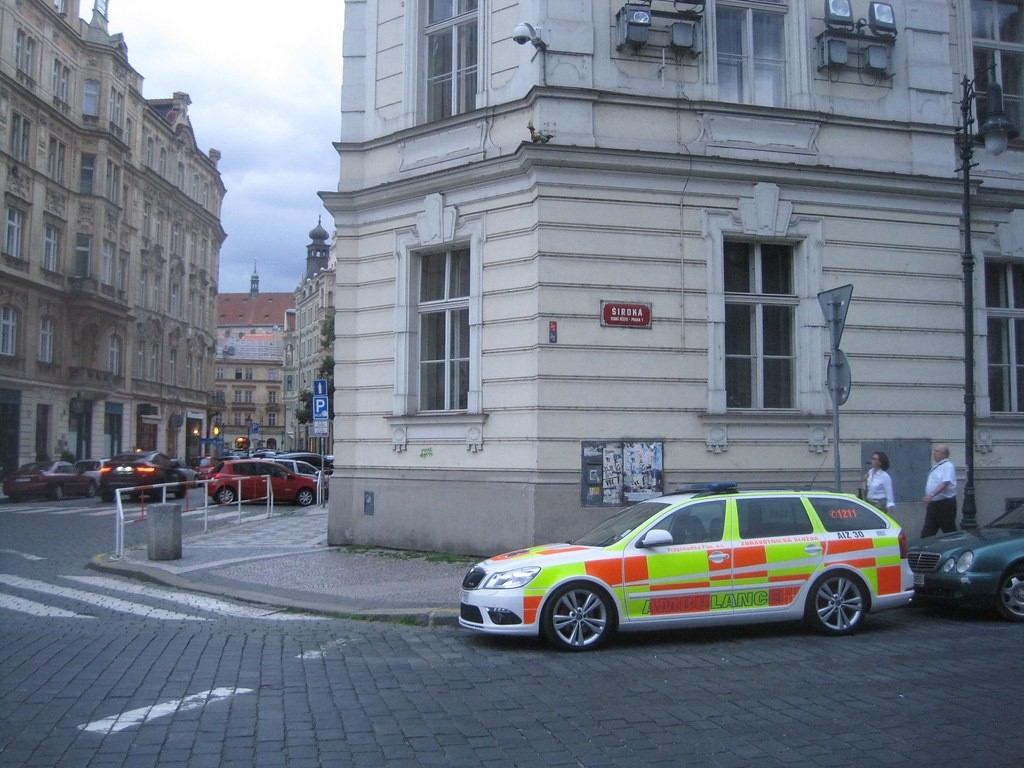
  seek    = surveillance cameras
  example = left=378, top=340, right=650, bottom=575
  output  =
left=512, top=26, right=531, bottom=44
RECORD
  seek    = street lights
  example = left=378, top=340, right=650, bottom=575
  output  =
left=244, top=417, right=253, bottom=458
left=949, top=60, right=1021, bottom=530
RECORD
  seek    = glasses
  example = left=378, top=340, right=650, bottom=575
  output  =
left=872, top=456, right=880, bottom=460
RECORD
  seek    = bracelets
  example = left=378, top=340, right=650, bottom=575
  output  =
left=929, top=492, right=935, bottom=497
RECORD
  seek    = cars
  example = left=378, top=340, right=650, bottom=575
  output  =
left=99, top=450, right=187, bottom=501
left=171, top=457, right=201, bottom=489
left=2, top=457, right=100, bottom=503
left=457, top=481, right=917, bottom=655
left=191, top=446, right=333, bottom=498
left=207, top=456, right=317, bottom=507
left=905, top=500, right=1024, bottom=626
left=74, top=458, right=111, bottom=485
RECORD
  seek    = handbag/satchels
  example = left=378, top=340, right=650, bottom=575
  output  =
left=859, top=487, right=868, bottom=501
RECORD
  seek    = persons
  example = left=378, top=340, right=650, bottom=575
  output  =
left=861, top=452, right=896, bottom=517
left=921, top=444, right=958, bottom=538
